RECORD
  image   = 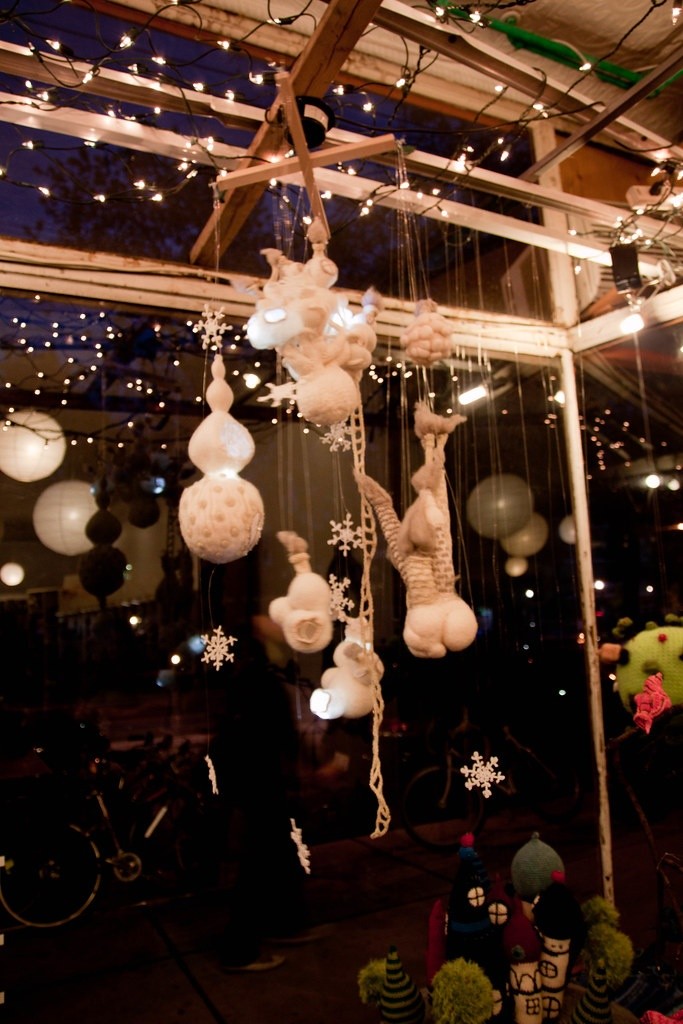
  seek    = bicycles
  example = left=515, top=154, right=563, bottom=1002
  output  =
left=401, top=705, right=586, bottom=855
left=0, top=732, right=252, bottom=930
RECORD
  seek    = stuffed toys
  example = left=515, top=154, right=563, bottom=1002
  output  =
left=268, top=530, right=334, bottom=655
left=398, top=299, right=456, bottom=367
left=601, top=625, right=683, bottom=736
left=358, top=831, right=632, bottom=1023
left=244, top=215, right=382, bottom=426
left=356, top=396, right=482, bottom=659
left=313, top=618, right=386, bottom=720
left=179, top=352, right=266, bottom=565
